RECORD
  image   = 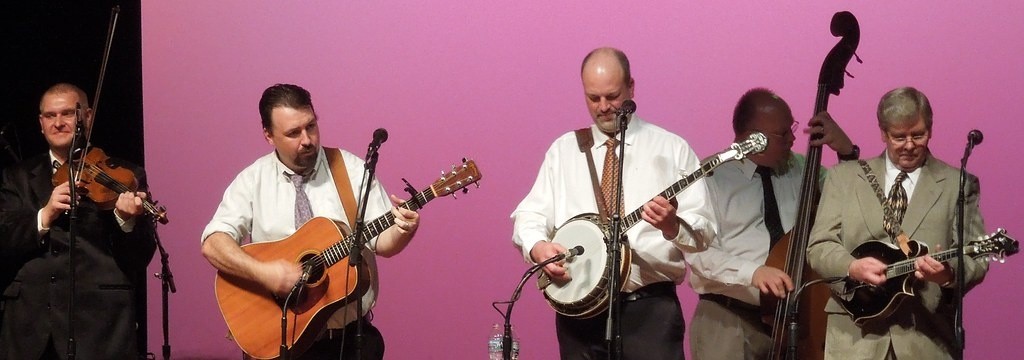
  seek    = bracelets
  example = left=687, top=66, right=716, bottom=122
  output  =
left=940, top=272, right=955, bottom=288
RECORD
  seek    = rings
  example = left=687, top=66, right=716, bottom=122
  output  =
left=404, top=222, right=409, bottom=229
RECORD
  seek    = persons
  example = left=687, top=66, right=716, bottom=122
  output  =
left=509, top=47, right=717, bottom=360
left=807, top=87, right=989, bottom=360
left=201, top=83, right=420, bottom=360
left=690, top=87, right=853, bottom=360
left=0, top=84, right=157, bottom=360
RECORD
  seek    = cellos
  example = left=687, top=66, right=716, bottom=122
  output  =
left=759, top=10, right=864, bottom=360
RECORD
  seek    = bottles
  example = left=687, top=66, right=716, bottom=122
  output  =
left=488, top=324, right=504, bottom=360
left=510, top=324, right=520, bottom=360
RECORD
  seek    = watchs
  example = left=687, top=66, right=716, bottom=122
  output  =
left=837, top=145, right=860, bottom=160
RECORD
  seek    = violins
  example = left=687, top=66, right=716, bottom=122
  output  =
left=52, top=146, right=169, bottom=225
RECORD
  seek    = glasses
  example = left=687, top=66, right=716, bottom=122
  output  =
left=884, top=129, right=929, bottom=145
left=744, top=122, right=798, bottom=140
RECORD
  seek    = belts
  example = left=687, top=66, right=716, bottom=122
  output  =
left=622, top=281, right=676, bottom=301
left=322, top=311, right=370, bottom=340
left=699, top=293, right=769, bottom=314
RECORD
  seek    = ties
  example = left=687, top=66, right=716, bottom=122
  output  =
left=602, top=136, right=626, bottom=223
left=759, top=164, right=785, bottom=250
left=883, top=170, right=907, bottom=245
left=291, top=173, right=314, bottom=232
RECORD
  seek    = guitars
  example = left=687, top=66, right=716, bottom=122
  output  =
left=536, top=133, right=769, bottom=319
left=835, top=227, right=1020, bottom=328
left=214, top=156, right=482, bottom=360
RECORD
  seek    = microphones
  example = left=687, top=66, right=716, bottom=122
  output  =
left=303, top=260, right=315, bottom=283
left=967, top=129, right=983, bottom=145
left=560, top=246, right=585, bottom=259
left=75, top=103, right=86, bottom=149
left=371, top=129, right=388, bottom=148
left=845, top=276, right=861, bottom=286
left=619, top=100, right=637, bottom=114
